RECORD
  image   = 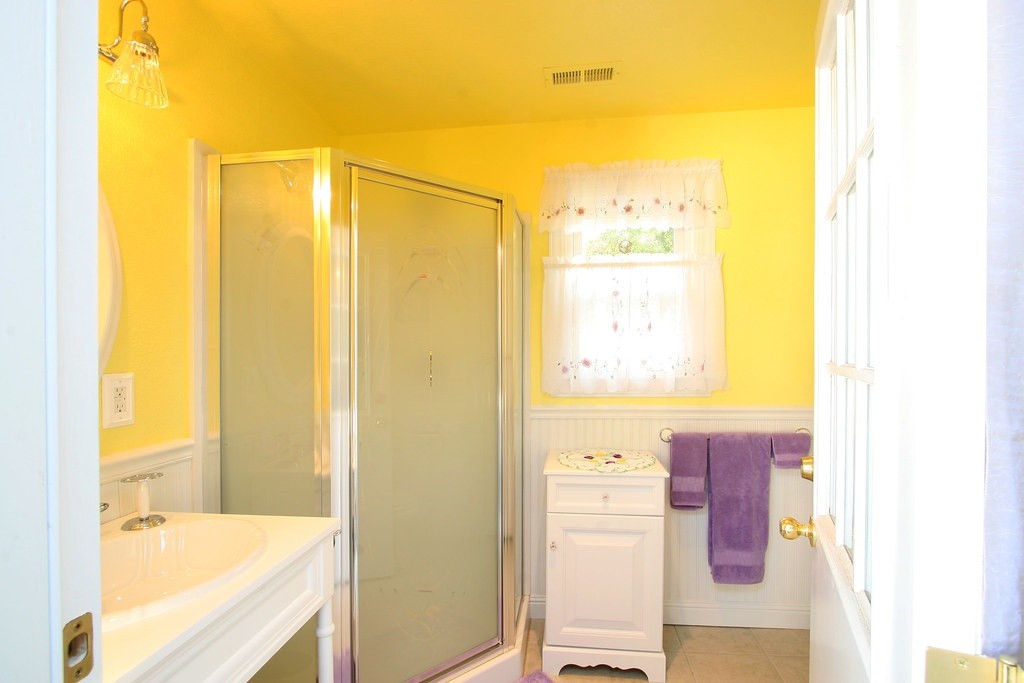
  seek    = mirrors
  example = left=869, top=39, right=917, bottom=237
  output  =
left=98, top=193, right=122, bottom=380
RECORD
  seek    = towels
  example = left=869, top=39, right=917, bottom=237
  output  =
left=671, top=433, right=708, bottom=510
left=772, top=433, right=810, bottom=469
left=707, top=435, right=772, bottom=586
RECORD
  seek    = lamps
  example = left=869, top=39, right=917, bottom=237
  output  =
left=98, top=0, right=168, bottom=110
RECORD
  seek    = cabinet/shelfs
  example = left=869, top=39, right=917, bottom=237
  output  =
left=541, top=451, right=670, bottom=683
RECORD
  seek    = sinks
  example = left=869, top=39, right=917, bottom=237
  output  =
left=100, top=512, right=340, bottom=683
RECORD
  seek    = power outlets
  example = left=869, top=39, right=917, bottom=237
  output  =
left=102, top=372, right=136, bottom=430
